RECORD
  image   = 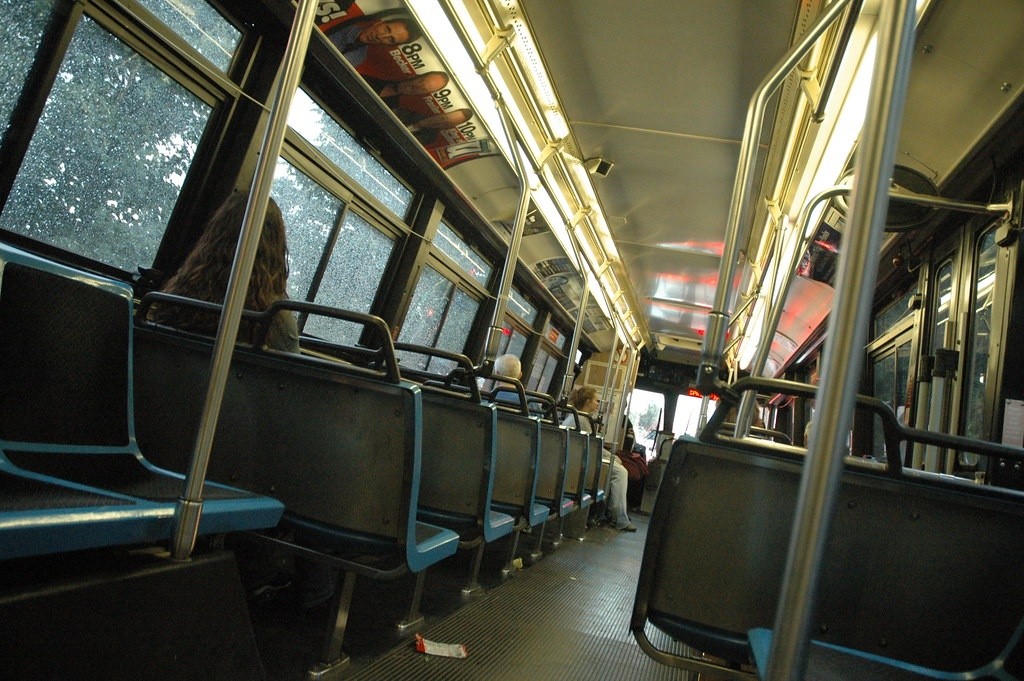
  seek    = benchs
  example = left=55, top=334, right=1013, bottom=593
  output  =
left=0, top=241, right=612, bottom=681
left=628, top=378, right=1024, bottom=681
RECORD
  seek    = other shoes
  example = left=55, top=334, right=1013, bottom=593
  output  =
left=610, top=518, right=638, bottom=532
left=605, top=511, right=632, bottom=523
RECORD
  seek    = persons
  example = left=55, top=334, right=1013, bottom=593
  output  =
left=149, top=188, right=300, bottom=355
left=562, top=385, right=637, bottom=532
left=544, top=276, right=569, bottom=290
left=622, top=401, right=635, bottom=452
left=493, top=353, right=542, bottom=418
left=804, top=421, right=813, bottom=448
left=316, top=17, right=421, bottom=84
left=338, top=71, right=449, bottom=124
left=716, top=393, right=764, bottom=435
left=368, top=108, right=473, bottom=155
